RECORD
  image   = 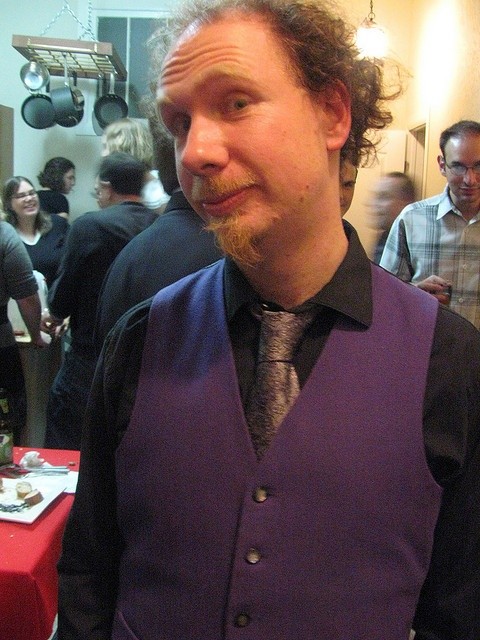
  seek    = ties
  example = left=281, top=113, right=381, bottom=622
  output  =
left=245, top=305, right=316, bottom=460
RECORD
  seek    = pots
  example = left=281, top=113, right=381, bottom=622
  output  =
left=92, top=70, right=130, bottom=137
left=71, top=69, right=84, bottom=107
left=21, top=58, right=49, bottom=95
left=50, top=55, right=76, bottom=119
left=57, top=109, right=84, bottom=128
left=21, top=89, right=56, bottom=129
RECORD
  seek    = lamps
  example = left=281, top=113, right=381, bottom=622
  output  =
left=353, top=0, right=392, bottom=60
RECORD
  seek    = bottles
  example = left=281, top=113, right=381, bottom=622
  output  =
left=0, top=388, right=13, bottom=467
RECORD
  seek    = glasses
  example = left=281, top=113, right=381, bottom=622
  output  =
left=9, top=190, right=38, bottom=201
left=442, top=157, right=480, bottom=176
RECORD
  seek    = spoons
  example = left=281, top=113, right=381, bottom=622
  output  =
left=0, top=463, right=70, bottom=479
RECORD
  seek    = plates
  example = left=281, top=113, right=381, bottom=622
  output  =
left=0, top=473, right=71, bottom=525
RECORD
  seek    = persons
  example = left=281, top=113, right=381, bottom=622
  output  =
left=90, top=100, right=224, bottom=368
left=57, top=0, right=480, bottom=640
left=43, top=152, right=160, bottom=452
left=0, top=176, right=70, bottom=451
left=336, top=153, right=357, bottom=217
left=35, top=157, right=75, bottom=221
left=370, top=172, right=416, bottom=265
left=0, top=221, right=41, bottom=446
left=100, top=118, right=172, bottom=219
left=379, top=121, right=480, bottom=329
